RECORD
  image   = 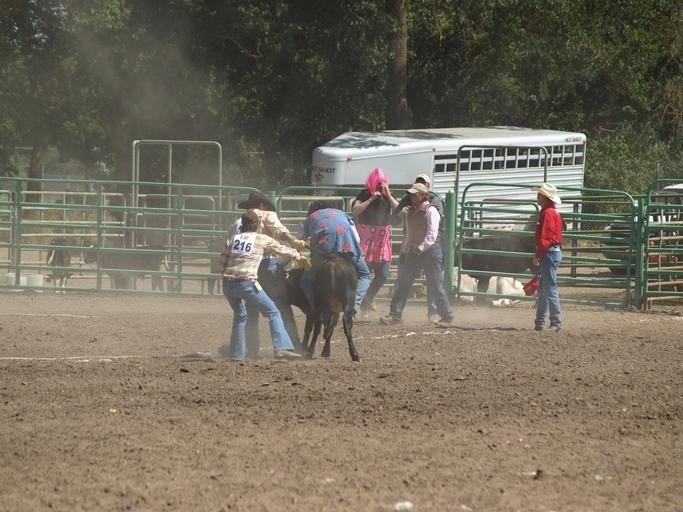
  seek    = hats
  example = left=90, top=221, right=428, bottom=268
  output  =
left=531, top=183, right=561, bottom=204
left=238, top=192, right=275, bottom=210
left=406, top=174, right=431, bottom=194
left=242, top=211, right=261, bottom=224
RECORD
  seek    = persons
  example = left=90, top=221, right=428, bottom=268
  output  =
left=379, top=183, right=455, bottom=326
left=219, top=211, right=311, bottom=363
left=291, top=198, right=372, bottom=316
left=351, top=166, right=399, bottom=313
left=392, top=174, right=444, bottom=323
left=225, top=188, right=308, bottom=357
left=531, top=182, right=565, bottom=332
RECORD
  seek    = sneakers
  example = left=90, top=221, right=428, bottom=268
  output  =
left=535, top=324, right=562, bottom=331
left=363, top=296, right=376, bottom=311
left=324, top=313, right=339, bottom=339
left=381, top=316, right=395, bottom=325
left=427, top=313, right=457, bottom=327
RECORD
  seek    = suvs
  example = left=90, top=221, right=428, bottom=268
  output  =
left=597, top=183, right=683, bottom=277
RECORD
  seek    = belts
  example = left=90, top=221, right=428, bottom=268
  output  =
left=230, top=279, right=250, bottom=282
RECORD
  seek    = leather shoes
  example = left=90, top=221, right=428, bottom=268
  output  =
left=275, top=349, right=303, bottom=361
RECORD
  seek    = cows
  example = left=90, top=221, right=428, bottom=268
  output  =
left=45, top=241, right=73, bottom=296
left=260, top=254, right=363, bottom=363
left=439, top=266, right=526, bottom=305
left=441, top=235, right=541, bottom=305
left=83, top=244, right=165, bottom=291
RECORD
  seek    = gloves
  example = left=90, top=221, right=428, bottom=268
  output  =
left=399, top=253, right=406, bottom=265
left=412, top=248, right=423, bottom=259
